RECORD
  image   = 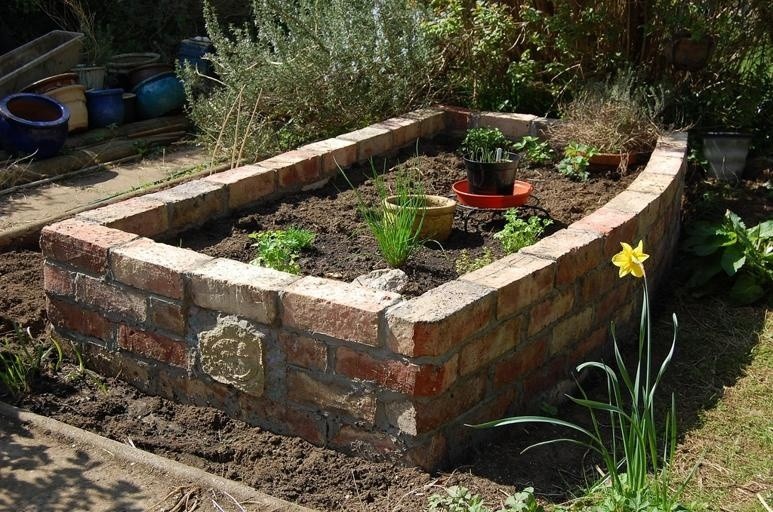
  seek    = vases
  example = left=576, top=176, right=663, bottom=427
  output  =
left=0, top=35, right=189, bottom=159
left=383, top=194, right=456, bottom=244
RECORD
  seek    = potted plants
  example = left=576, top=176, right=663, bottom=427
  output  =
left=462, top=144, right=521, bottom=196
left=547, top=92, right=660, bottom=171
left=696, top=63, right=767, bottom=182
